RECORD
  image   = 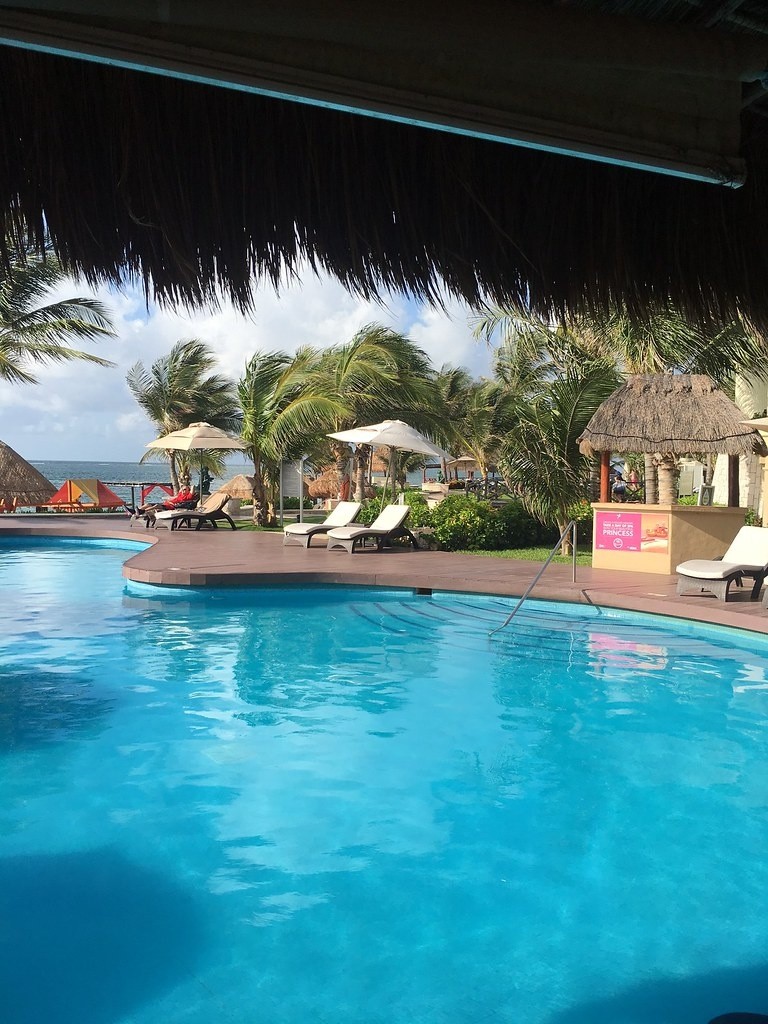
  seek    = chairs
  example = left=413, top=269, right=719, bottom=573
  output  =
left=130, top=493, right=202, bottom=528
left=155, top=492, right=237, bottom=531
left=676, top=525, right=768, bottom=602
left=326, top=505, right=418, bottom=554
left=283, top=501, right=362, bottom=549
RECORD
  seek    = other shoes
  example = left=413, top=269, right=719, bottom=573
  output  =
left=135, top=506, right=145, bottom=516
left=122, top=504, right=135, bottom=517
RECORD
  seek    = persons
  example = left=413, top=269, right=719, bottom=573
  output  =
left=121, top=485, right=192, bottom=519
left=628, top=469, right=639, bottom=491
left=612, top=475, right=628, bottom=503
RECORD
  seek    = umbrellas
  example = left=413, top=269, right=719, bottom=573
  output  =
left=448, top=456, right=481, bottom=480
left=146, top=422, right=257, bottom=507
left=325, top=420, right=455, bottom=518
left=573, top=373, right=768, bottom=508
